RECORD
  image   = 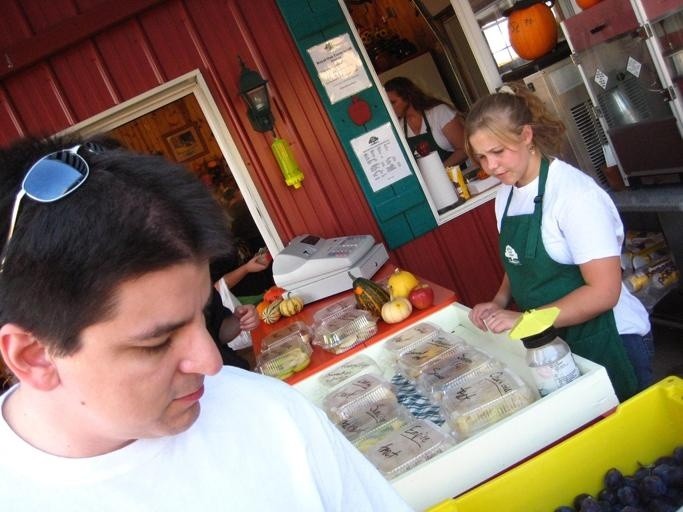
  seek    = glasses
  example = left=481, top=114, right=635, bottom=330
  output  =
left=0, top=131, right=121, bottom=277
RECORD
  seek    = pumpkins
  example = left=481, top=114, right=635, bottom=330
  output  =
left=381, top=298, right=413, bottom=323
left=264, top=285, right=285, bottom=302
left=387, top=268, right=418, bottom=299
left=279, top=292, right=303, bottom=317
left=348, top=271, right=390, bottom=317
left=256, top=301, right=270, bottom=320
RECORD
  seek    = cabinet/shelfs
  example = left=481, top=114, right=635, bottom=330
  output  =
left=610, top=185, right=683, bottom=328
left=251, top=263, right=460, bottom=387
left=425, top=375, right=683, bottom=512
left=291, top=301, right=620, bottom=512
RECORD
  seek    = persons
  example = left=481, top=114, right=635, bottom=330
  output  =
left=209, top=245, right=272, bottom=298
left=465, top=83, right=655, bottom=404
left=384, top=75, right=471, bottom=174
left=204, top=282, right=257, bottom=372
left=1, top=137, right=417, bottom=512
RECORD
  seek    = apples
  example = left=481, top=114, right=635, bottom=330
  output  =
left=409, top=282, right=434, bottom=310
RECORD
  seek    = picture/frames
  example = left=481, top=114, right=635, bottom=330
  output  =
left=159, top=121, right=208, bottom=164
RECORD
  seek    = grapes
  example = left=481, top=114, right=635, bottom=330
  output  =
left=553, top=443, right=683, bottom=512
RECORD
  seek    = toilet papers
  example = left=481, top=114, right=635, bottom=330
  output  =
left=416, top=151, right=458, bottom=210
